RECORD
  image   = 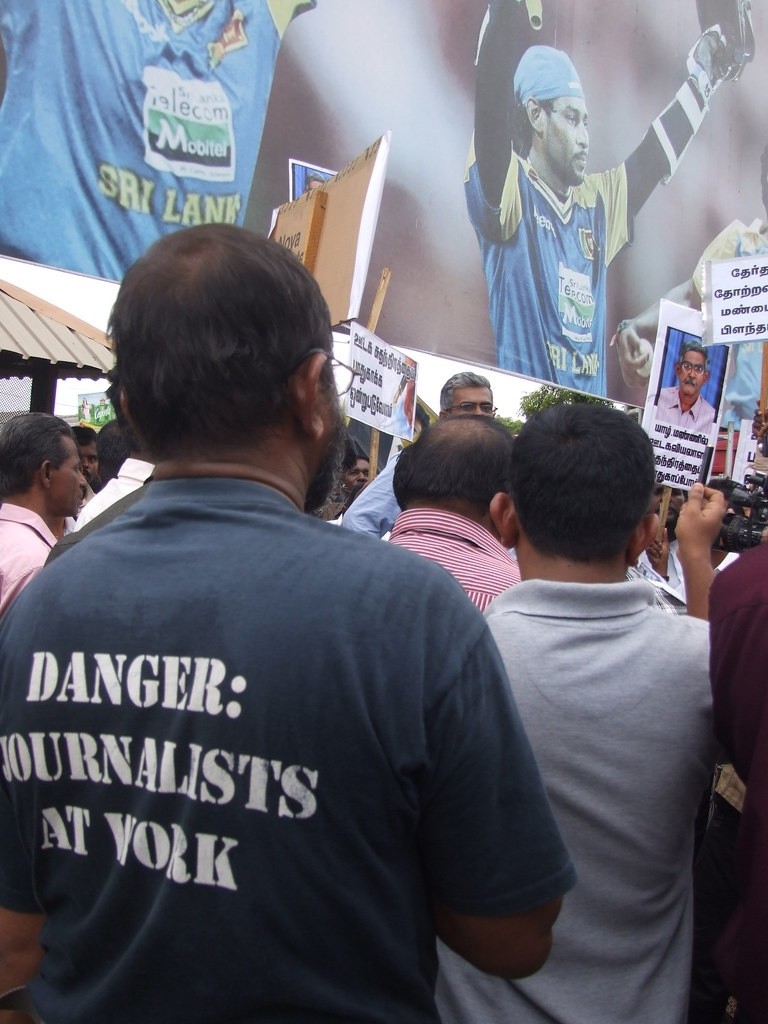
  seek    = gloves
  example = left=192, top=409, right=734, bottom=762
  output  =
left=687, top=24, right=731, bottom=105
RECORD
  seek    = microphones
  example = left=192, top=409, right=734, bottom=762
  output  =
left=707, top=479, right=754, bottom=508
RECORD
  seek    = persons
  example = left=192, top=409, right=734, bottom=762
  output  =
left=656, top=339, right=715, bottom=434
left=0, top=364, right=156, bottom=620
left=320, top=372, right=522, bottom=612
left=437, top=405, right=768, bottom=1024
left=614, top=145, right=768, bottom=430
left=304, top=172, right=325, bottom=193
left=455, top=0, right=734, bottom=398
left=0, top=224, right=580, bottom=1024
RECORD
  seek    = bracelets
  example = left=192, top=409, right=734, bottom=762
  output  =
left=610, top=319, right=630, bottom=347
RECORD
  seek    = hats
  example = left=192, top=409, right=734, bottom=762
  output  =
left=513, top=45, right=584, bottom=110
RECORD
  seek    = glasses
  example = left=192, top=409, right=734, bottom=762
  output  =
left=680, top=361, right=706, bottom=376
left=446, top=402, right=498, bottom=416
left=289, top=348, right=361, bottom=397
